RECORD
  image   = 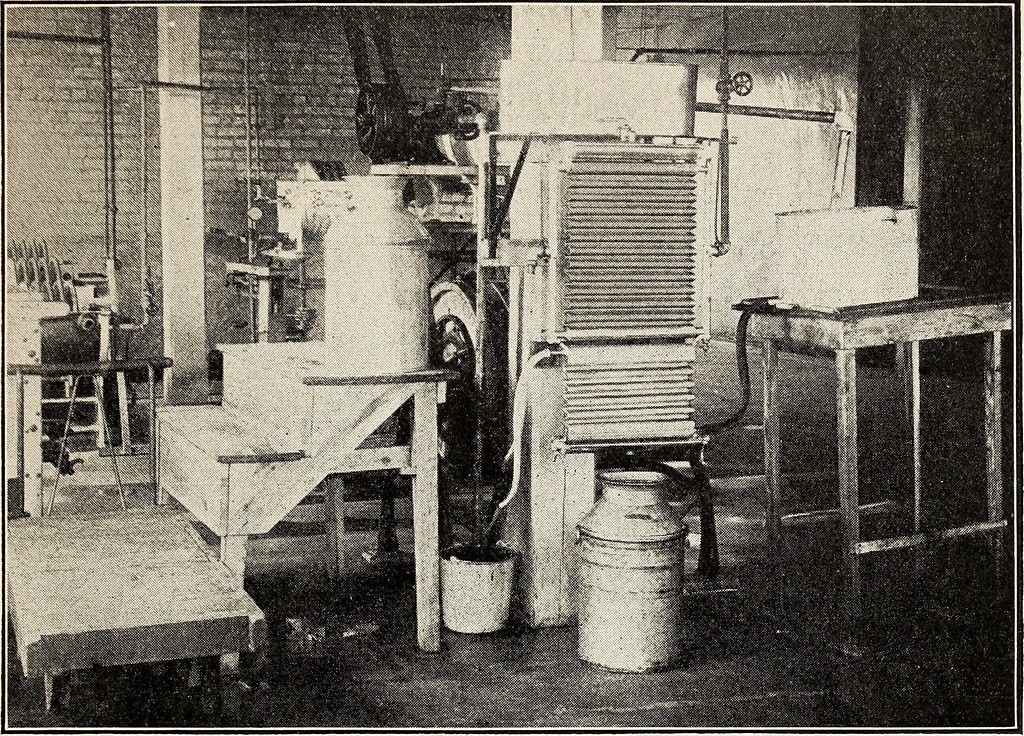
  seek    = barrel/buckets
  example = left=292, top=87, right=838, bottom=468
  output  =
left=577, top=472, right=686, bottom=671
left=438, top=544, right=516, bottom=633
